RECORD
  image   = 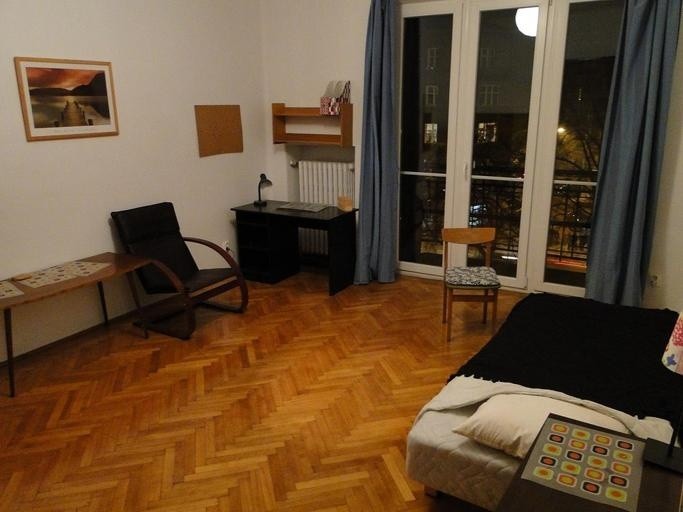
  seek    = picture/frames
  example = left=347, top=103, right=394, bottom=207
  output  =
left=13, top=57, right=119, bottom=142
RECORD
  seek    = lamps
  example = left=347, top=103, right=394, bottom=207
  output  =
left=253, top=172, right=273, bottom=207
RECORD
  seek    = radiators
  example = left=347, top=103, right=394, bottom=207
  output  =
left=289, top=159, right=354, bottom=255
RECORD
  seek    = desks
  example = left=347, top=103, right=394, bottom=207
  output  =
left=0, top=251, right=153, bottom=397
left=229, top=199, right=358, bottom=296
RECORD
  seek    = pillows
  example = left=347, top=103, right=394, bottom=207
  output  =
left=451, top=393, right=632, bottom=460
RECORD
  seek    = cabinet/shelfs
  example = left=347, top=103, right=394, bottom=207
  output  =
left=271, top=103, right=353, bottom=148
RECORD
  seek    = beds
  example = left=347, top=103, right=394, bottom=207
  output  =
left=405, top=293, right=683, bottom=512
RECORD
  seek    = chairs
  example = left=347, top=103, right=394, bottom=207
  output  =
left=110, top=202, right=248, bottom=340
left=441, top=227, right=501, bottom=343
